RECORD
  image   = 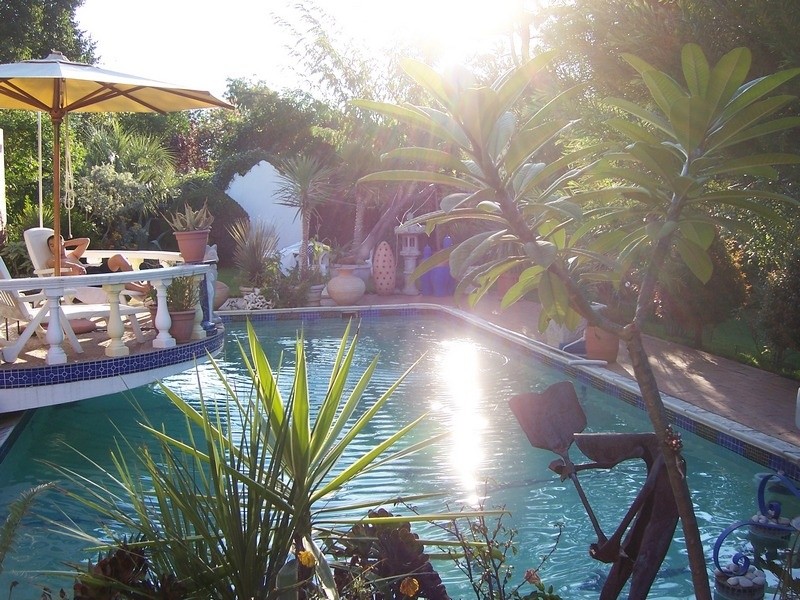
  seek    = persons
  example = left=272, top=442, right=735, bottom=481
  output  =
left=47, top=234, right=154, bottom=292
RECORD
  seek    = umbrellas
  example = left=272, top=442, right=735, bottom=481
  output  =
left=0, top=53, right=236, bottom=276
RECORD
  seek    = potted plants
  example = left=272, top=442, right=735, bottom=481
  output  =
left=225, top=219, right=285, bottom=297
left=142, top=271, right=202, bottom=343
left=292, top=264, right=324, bottom=307
left=580, top=252, right=632, bottom=363
left=157, top=196, right=214, bottom=261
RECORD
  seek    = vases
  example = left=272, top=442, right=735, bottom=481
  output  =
left=327, top=267, right=366, bottom=305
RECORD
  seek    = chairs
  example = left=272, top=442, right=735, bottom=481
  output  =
left=0, top=256, right=150, bottom=344
left=23, top=227, right=148, bottom=319
